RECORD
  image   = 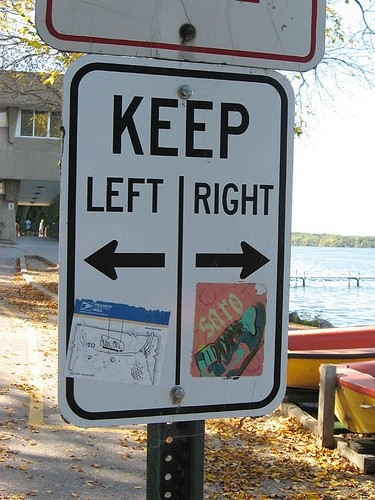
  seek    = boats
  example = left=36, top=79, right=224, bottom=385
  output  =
left=284, top=324, right=375, bottom=389
left=332, top=361, right=375, bottom=435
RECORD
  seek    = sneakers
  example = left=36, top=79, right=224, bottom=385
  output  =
left=195, top=303, right=265, bottom=377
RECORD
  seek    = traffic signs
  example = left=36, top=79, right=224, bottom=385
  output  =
left=55, top=55, right=296, bottom=430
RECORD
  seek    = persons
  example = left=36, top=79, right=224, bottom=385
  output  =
left=24, top=217, right=31, bottom=236
left=15, top=221, right=20, bottom=237
